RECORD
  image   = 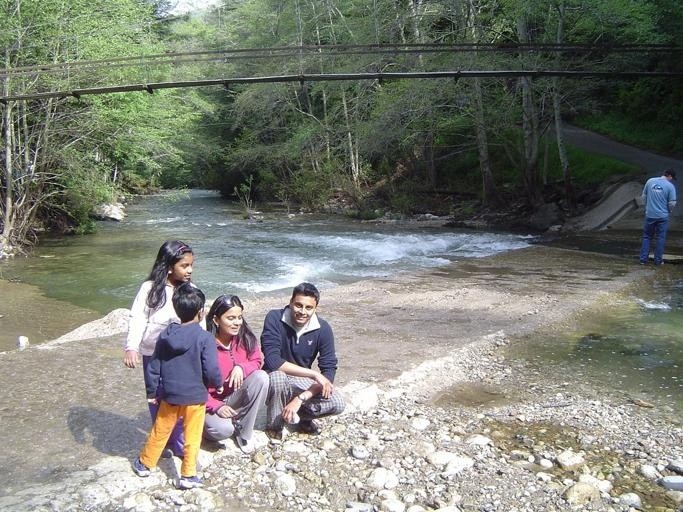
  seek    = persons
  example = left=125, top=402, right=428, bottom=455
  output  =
left=260, top=282, right=346, bottom=439
left=639, top=168, right=677, bottom=266
left=123, top=240, right=269, bottom=489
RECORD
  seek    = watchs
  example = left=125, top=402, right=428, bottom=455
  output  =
left=298, top=394, right=307, bottom=403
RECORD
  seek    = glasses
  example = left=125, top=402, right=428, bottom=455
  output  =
left=214, top=295, right=241, bottom=312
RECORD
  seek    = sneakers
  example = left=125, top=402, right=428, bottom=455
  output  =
left=179, top=476, right=204, bottom=488
left=235, top=435, right=254, bottom=455
left=299, top=419, right=318, bottom=434
left=264, top=426, right=283, bottom=449
left=639, top=260, right=665, bottom=266
left=132, top=457, right=150, bottom=477
left=161, top=448, right=173, bottom=458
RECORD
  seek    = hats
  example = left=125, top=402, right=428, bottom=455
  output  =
left=664, top=167, right=677, bottom=181
